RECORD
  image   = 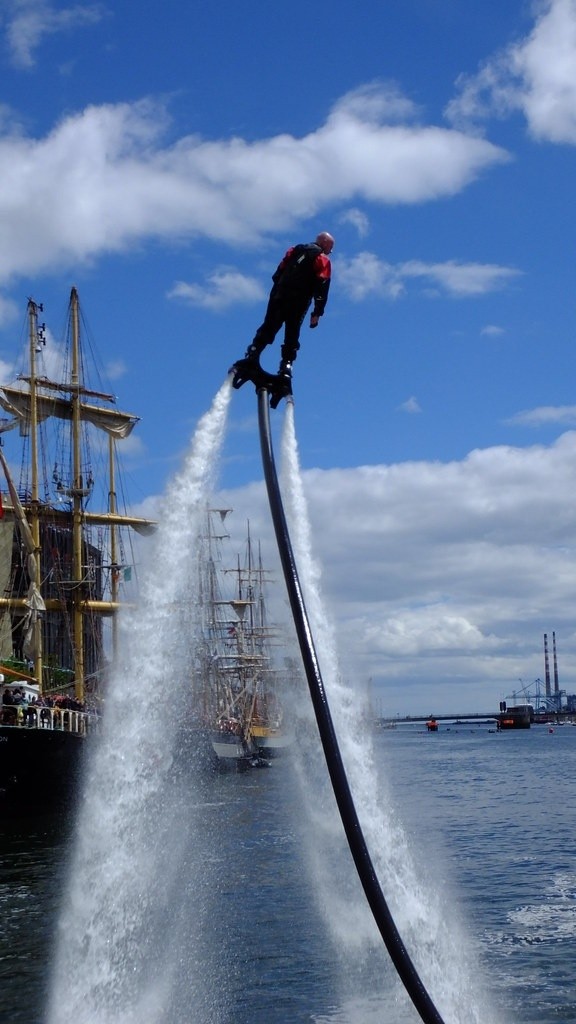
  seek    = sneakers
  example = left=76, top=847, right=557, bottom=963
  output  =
left=248, top=345, right=261, bottom=360
left=279, top=360, right=293, bottom=377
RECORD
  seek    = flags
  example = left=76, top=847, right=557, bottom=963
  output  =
left=124, top=567, right=131, bottom=581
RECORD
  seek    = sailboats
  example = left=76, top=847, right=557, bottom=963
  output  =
left=0, top=284, right=299, bottom=775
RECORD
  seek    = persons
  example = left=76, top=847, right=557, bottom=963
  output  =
left=2, top=686, right=90, bottom=729
left=245, top=232, right=335, bottom=379
